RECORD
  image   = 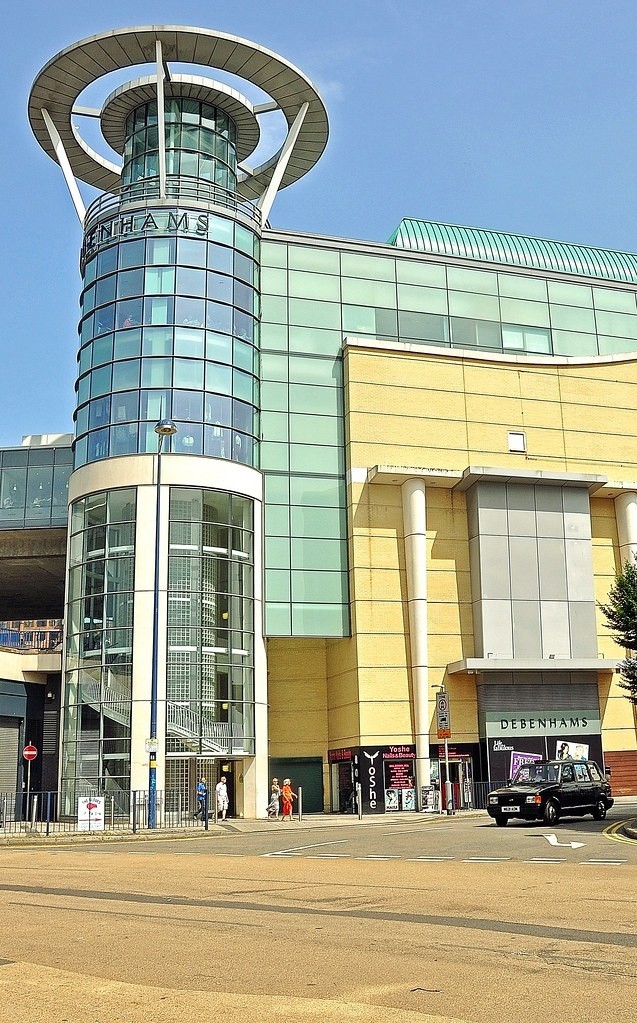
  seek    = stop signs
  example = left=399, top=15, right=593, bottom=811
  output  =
left=22, top=745, right=37, bottom=761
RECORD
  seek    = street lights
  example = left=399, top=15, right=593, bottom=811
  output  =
left=432, top=684, right=453, bottom=815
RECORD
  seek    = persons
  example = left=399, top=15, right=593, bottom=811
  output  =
left=558, top=743, right=586, bottom=762
left=265, top=778, right=281, bottom=820
left=212, top=777, right=229, bottom=822
left=192, top=778, right=208, bottom=821
left=281, top=778, right=297, bottom=822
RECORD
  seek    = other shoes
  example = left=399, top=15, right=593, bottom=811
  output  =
left=290, top=818, right=296, bottom=821
left=194, top=815, right=197, bottom=820
left=222, top=818, right=227, bottom=821
left=282, top=819, right=286, bottom=822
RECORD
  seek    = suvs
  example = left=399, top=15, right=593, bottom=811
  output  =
left=487, top=759, right=614, bottom=828
